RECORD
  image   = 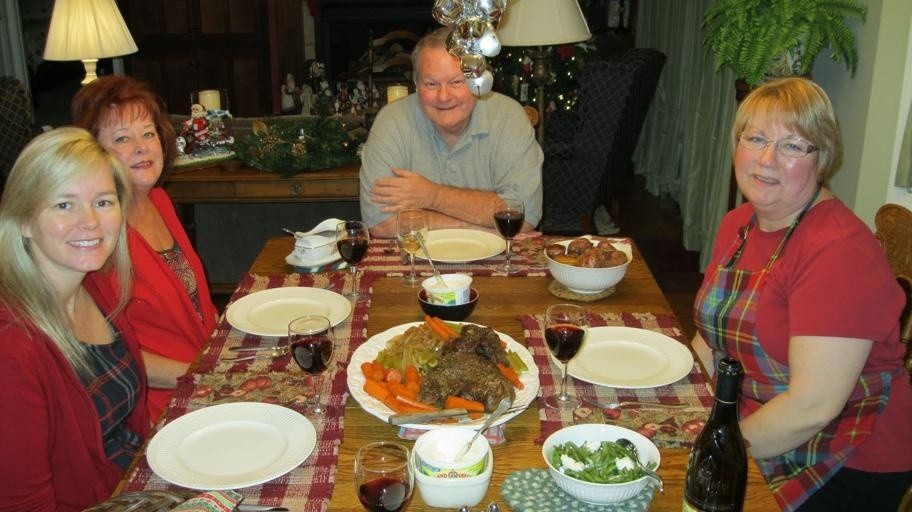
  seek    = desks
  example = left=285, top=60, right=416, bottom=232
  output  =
left=161, top=115, right=374, bottom=294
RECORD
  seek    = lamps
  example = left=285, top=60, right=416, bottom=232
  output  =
left=496, top=1, right=593, bottom=146
left=43, top=0, right=139, bottom=87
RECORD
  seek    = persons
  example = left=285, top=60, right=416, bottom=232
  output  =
left=359, top=26, right=545, bottom=241
left=173, top=103, right=237, bottom=166
left=70, top=76, right=219, bottom=424
left=0, top=126, right=152, bottom=512
left=691, top=77, right=912, bottom=512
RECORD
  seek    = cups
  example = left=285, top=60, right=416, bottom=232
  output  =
left=421, top=274, right=472, bottom=302
left=295, top=234, right=333, bottom=261
left=380, top=82, right=410, bottom=105
left=416, top=429, right=490, bottom=476
left=190, top=88, right=231, bottom=114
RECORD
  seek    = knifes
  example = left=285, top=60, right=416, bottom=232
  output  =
left=228, top=346, right=288, bottom=352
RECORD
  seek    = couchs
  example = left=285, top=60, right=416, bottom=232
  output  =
left=542, top=46, right=667, bottom=229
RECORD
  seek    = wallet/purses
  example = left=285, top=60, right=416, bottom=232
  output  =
left=82, top=490, right=186, bottom=512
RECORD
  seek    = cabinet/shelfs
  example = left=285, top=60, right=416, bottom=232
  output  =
left=113, top=0, right=324, bottom=120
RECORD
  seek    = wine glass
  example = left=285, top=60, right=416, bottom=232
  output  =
left=336, top=220, right=369, bottom=304
left=288, top=315, right=336, bottom=420
left=492, top=198, right=525, bottom=271
left=544, top=303, right=592, bottom=412
left=396, top=208, right=428, bottom=288
left=353, top=441, right=414, bottom=512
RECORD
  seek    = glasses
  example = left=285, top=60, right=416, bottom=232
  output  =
left=737, top=131, right=821, bottom=158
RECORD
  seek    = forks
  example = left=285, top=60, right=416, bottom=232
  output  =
left=581, top=393, right=688, bottom=411
left=388, top=405, right=529, bottom=427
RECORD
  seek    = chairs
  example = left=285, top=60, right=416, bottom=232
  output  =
left=366, top=27, right=431, bottom=109
left=872, top=203, right=912, bottom=367
left=1, top=76, right=33, bottom=195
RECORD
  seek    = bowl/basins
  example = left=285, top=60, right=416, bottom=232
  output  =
left=543, top=240, right=633, bottom=294
left=411, top=429, right=493, bottom=507
left=542, top=423, right=661, bottom=505
left=417, top=288, right=479, bottom=320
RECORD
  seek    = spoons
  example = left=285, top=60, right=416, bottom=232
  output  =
left=384, top=239, right=395, bottom=253
left=617, top=437, right=663, bottom=486
left=221, top=350, right=289, bottom=364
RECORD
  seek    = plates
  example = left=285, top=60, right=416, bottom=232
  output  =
left=147, top=401, right=318, bottom=492
left=415, top=228, right=506, bottom=263
left=550, top=326, right=694, bottom=389
left=346, top=319, right=540, bottom=429
left=284, top=252, right=341, bottom=267
left=226, top=286, right=351, bottom=337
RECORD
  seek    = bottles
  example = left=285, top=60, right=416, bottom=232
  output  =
left=681, top=357, right=749, bottom=512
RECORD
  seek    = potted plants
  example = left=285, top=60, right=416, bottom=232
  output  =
left=698, top=0, right=868, bottom=214
